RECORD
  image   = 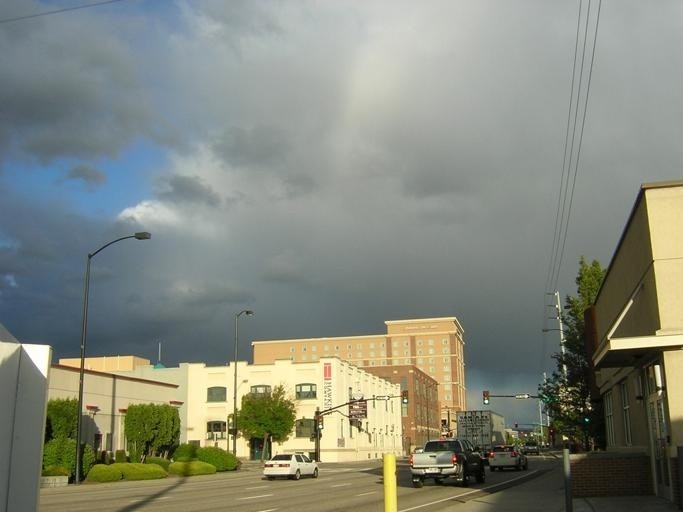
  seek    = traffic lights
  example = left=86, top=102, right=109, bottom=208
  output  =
left=349, top=418, right=362, bottom=426
left=515, top=424, right=518, bottom=428
left=482, top=390, right=490, bottom=405
left=403, top=391, right=408, bottom=404
left=318, top=415, right=324, bottom=430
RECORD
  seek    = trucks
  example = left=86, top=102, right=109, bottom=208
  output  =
left=456, top=410, right=508, bottom=459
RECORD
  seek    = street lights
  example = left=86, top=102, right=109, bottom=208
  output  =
left=233, top=309, right=254, bottom=457
left=75, top=232, right=151, bottom=486
left=542, top=328, right=570, bottom=336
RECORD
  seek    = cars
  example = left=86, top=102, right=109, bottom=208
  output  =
left=263, top=454, right=319, bottom=480
left=408, top=441, right=539, bottom=488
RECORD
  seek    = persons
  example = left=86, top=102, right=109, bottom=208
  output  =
left=566, top=437, right=575, bottom=454
